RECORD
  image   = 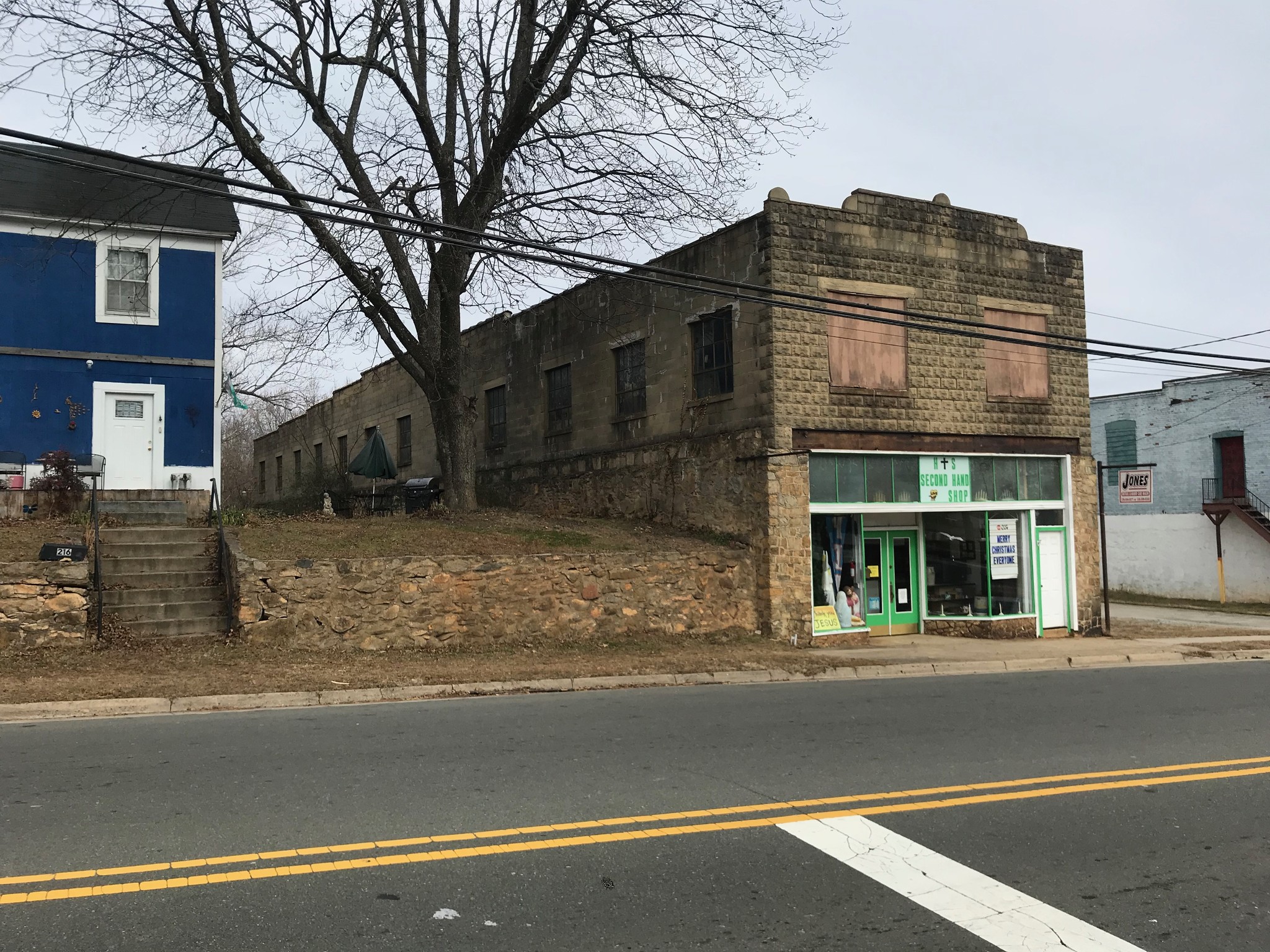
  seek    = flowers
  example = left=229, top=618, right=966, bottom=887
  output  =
left=32, top=409, right=40, bottom=419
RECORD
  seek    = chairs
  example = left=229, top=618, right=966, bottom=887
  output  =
left=324, top=489, right=361, bottom=519
left=373, top=484, right=410, bottom=518
left=318, top=492, right=354, bottom=519
left=75, top=454, right=106, bottom=489
left=0, top=450, right=27, bottom=491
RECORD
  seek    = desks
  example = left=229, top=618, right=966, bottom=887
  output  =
left=355, top=492, right=393, bottom=518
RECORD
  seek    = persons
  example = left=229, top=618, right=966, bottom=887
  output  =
left=844, top=586, right=860, bottom=615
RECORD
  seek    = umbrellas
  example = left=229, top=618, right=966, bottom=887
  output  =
left=348, top=425, right=398, bottom=516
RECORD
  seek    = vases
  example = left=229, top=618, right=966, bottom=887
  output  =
left=8, top=475, right=24, bottom=490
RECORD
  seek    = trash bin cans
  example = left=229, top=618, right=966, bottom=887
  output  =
left=403, top=477, right=440, bottom=514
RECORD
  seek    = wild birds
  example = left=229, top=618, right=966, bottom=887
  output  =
left=31, top=382, right=62, bottom=413
